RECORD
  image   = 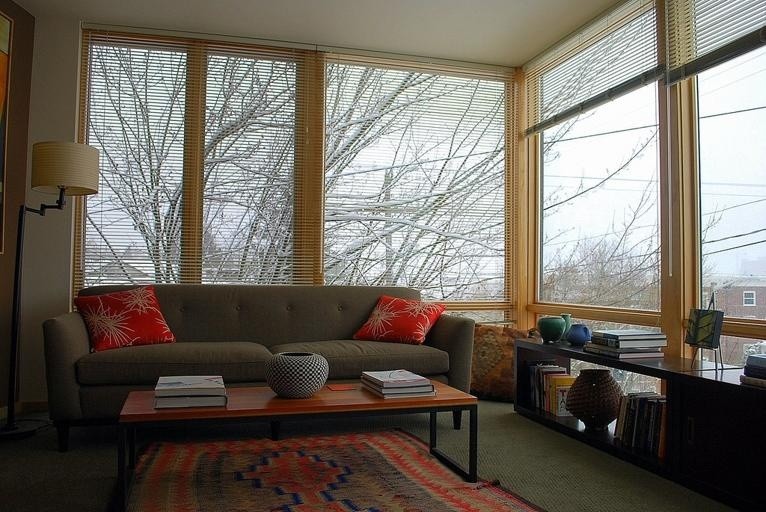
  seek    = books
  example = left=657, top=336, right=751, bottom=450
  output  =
left=525, top=358, right=578, bottom=419
left=361, top=382, right=436, bottom=400
left=743, top=364, right=766, bottom=382
left=154, top=394, right=229, bottom=409
left=612, top=391, right=667, bottom=459
left=747, top=354, right=765, bottom=368
left=582, top=329, right=669, bottom=359
left=684, top=308, right=725, bottom=348
left=362, top=368, right=431, bottom=387
left=739, top=375, right=766, bottom=388
left=360, top=375, right=434, bottom=395
left=155, top=377, right=226, bottom=396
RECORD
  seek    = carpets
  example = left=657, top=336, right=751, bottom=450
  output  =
left=122, top=425, right=554, bottom=511
left=420, top=468, right=423, bottom=472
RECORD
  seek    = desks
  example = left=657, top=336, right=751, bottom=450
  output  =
left=512, top=338, right=766, bottom=512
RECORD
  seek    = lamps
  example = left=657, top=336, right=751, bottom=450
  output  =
left=0, top=138, right=101, bottom=441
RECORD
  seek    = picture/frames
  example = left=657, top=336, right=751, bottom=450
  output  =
left=0, top=8, right=17, bottom=261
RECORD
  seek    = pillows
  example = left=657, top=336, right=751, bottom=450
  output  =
left=352, top=293, right=448, bottom=346
left=468, top=322, right=529, bottom=404
left=73, top=283, right=177, bottom=354
